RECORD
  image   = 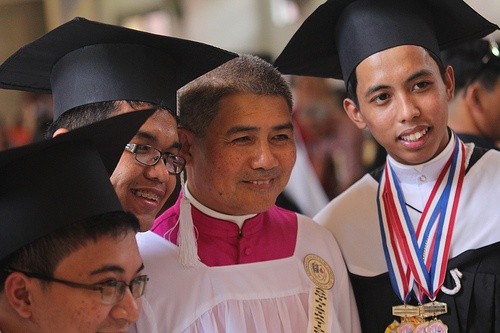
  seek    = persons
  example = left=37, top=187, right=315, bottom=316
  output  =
left=0, top=0, right=500, bottom=333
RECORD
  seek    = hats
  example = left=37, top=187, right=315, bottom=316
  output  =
left=1, top=17, right=241, bottom=119
left=0, top=109, right=157, bottom=253
left=272, top=0, right=500, bottom=90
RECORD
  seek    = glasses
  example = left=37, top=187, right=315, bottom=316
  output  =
left=124, top=143, right=186, bottom=174
left=11, top=269, right=149, bottom=302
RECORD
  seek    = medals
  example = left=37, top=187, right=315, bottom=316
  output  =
left=388, top=305, right=420, bottom=333
left=413, top=302, right=449, bottom=333
